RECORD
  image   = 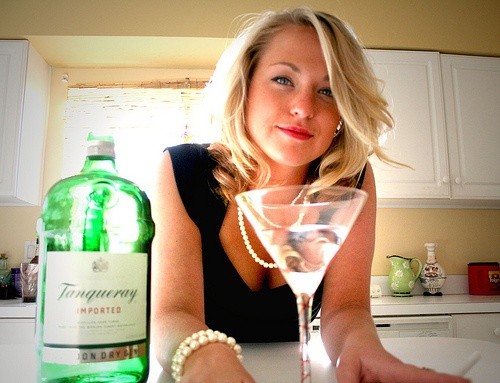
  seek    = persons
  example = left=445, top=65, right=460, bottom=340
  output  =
left=146, top=8, right=471, bottom=383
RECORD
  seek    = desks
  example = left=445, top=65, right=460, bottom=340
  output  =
left=1, top=337, right=500, bottom=383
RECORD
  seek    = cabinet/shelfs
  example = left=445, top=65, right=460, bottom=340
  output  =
left=360, top=49, right=500, bottom=210
left=0, top=40, right=51, bottom=206
left=451, top=314, right=500, bottom=343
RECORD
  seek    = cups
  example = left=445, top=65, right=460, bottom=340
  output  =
left=467, top=261, right=500, bottom=296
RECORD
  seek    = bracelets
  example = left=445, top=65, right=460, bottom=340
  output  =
left=172, top=328, right=242, bottom=383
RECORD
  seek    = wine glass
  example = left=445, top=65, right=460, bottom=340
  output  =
left=235, top=184, right=369, bottom=383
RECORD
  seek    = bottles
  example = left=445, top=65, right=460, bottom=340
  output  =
left=421, top=242, right=447, bottom=295
left=35, top=132, right=155, bottom=383
left=1, top=253, right=12, bottom=285
left=10, top=267, right=22, bottom=298
left=23, top=255, right=37, bottom=302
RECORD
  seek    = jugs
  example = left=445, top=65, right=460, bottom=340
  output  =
left=386, top=253, right=423, bottom=297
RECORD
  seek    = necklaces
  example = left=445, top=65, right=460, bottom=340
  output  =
left=236, top=196, right=313, bottom=271
